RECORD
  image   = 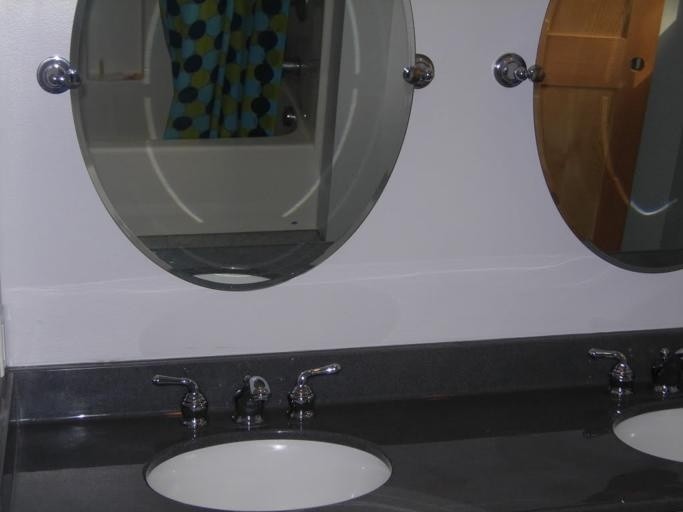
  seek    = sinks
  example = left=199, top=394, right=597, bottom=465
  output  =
left=144, top=424, right=393, bottom=512
left=611, top=398, right=683, bottom=463
left=191, top=267, right=281, bottom=284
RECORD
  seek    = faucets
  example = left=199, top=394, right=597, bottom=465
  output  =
left=227, top=372, right=272, bottom=426
left=649, top=347, right=683, bottom=399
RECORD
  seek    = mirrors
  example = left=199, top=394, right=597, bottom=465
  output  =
left=494, top=0, right=683, bottom=273
left=34, top=0, right=434, bottom=292
